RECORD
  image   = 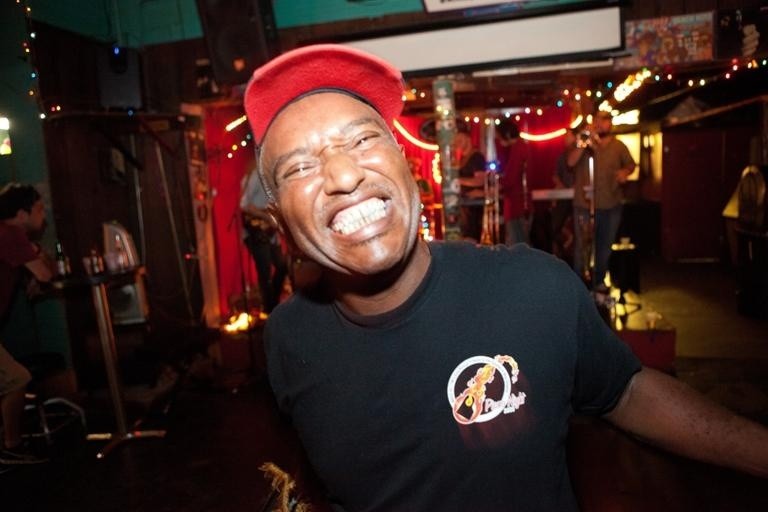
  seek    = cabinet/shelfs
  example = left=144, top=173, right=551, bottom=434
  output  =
left=54, top=240, right=68, bottom=280
left=83, top=255, right=104, bottom=276
left=113, top=234, right=130, bottom=270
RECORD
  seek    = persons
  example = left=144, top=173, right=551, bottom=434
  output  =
left=454, top=118, right=492, bottom=248
left=463, top=121, right=535, bottom=246
left=716, top=8, right=761, bottom=59
left=239, top=159, right=289, bottom=314
left=625, top=15, right=713, bottom=62
left=565, top=110, right=638, bottom=294
left=235, top=43, right=767, bottom=510
left=0, top=184, right=57, bottom=463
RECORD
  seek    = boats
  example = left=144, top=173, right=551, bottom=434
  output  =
left=97, top=46, right=143, bottom=110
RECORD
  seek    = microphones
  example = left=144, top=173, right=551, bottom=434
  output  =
left=41, top=262, right=166, bottom=463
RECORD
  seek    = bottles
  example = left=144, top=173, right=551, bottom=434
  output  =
left=244, top=43, right=406, bottom=147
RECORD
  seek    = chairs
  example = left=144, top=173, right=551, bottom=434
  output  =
left=0, top=441, right=51, bottom=465
